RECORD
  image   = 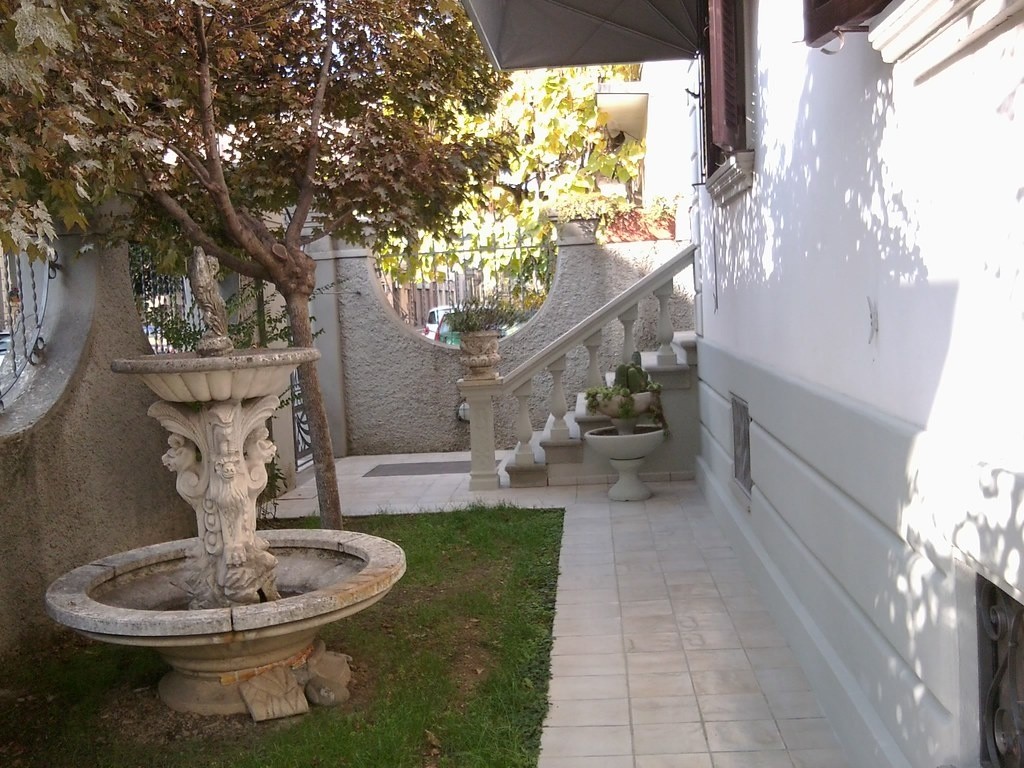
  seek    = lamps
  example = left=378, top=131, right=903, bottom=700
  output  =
left=709, top=141, right=734, bottom=167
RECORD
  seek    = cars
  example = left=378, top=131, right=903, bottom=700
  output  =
left=422, top=303, right=508, bottom=349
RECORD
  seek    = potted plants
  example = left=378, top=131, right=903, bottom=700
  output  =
left=446, top=289, right=527, bottom=383
left=585, top=354, right=671, bottom=501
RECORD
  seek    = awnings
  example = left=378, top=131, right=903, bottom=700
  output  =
left=458, top=1, right=704, bottom=72
